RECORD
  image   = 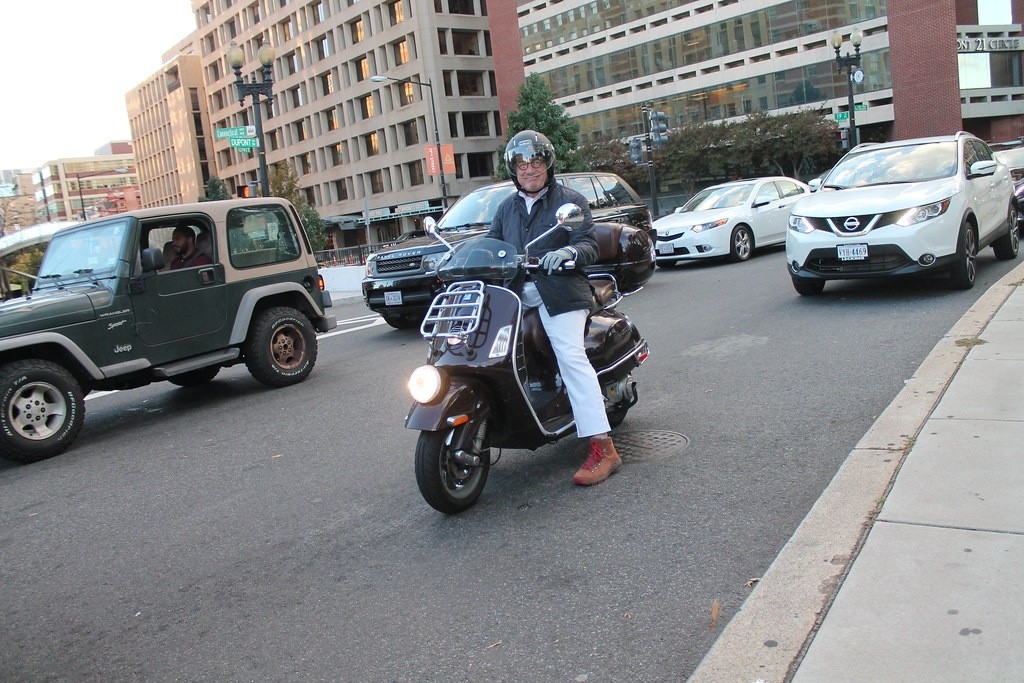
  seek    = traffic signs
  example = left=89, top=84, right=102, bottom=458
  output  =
left=215, top=125, right=261, bottom=153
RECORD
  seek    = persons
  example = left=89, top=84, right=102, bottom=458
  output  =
left=170, top=226, right=213, bottom=270
left=451, top=129, right=622, bottom=485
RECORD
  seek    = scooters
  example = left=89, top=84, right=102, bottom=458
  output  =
left=404, top=202, right=651, bottom=514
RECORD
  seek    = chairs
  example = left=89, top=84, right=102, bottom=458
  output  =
left=158, top=242, right=180, bottom=273
left=196, top=231, right=213, bottom=263
left=141, top=247, right=166, bottom=277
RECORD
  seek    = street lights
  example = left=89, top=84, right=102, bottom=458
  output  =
left=226, top=37, right=276, bottom=196
left=831, top=28, right=864, bottom=149
left=372, top=76, right=449, bottom=211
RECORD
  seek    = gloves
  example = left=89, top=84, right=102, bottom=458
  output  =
left=537, top=248, right=576, bottom=276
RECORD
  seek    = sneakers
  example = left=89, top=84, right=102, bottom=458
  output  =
left=573, top=436, right=623, bottom=484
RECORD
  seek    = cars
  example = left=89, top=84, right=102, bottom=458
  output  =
left=653, top=176, right=814, bottom=269
left=989, top=138, right=1024, bottom=222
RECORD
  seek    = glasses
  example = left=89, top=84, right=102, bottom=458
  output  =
left=514, top=157, right=549, bottom=170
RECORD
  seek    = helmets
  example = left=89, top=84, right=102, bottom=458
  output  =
left=503, top=130, right=556, bottom=186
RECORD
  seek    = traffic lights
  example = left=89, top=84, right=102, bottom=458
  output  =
left=649, top=110, right=668, bottom=145
left=834, top=129, right=849, bottom=151
left=855, top=127, right=865, bottom=146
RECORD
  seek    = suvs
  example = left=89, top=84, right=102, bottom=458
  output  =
left=785, top=129, right=1020, bottom=296
left=0, top=196, right=338, bottom=463
left=361, top=172, right=652, bottom=329
